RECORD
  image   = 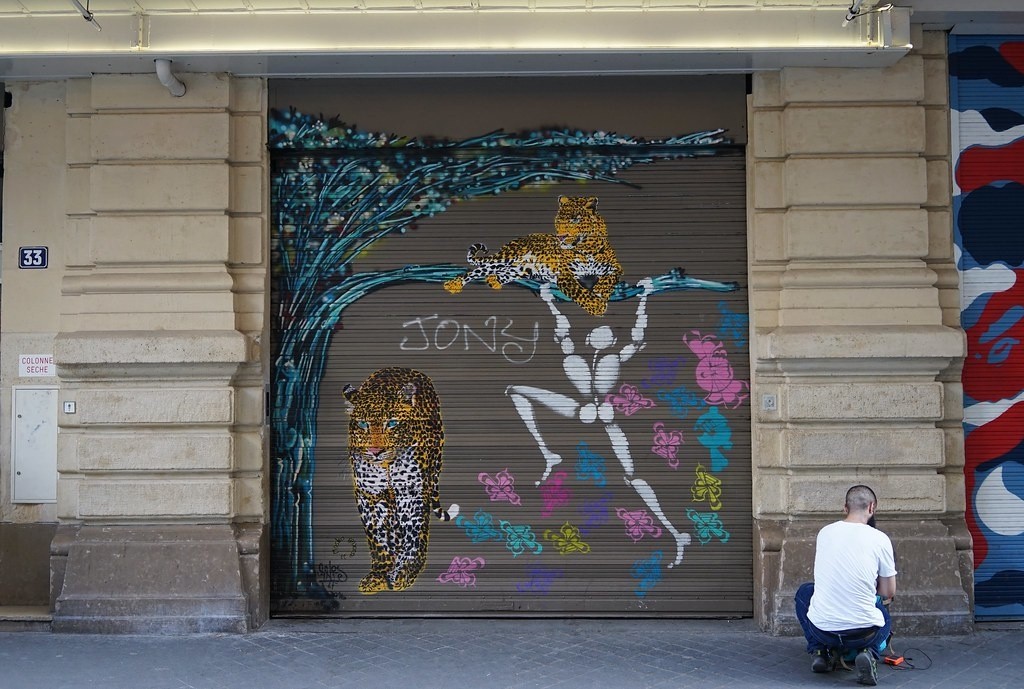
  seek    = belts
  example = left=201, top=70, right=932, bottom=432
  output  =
left=818, top=626, right=879, bottom=640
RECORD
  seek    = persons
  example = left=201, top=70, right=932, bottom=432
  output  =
left=795, top=485, right=897, bottom=686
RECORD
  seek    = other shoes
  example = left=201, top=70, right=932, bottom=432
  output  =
left=855, top=651, right=877, bottom=685
left=812, top=650, right=832, bottom=672
